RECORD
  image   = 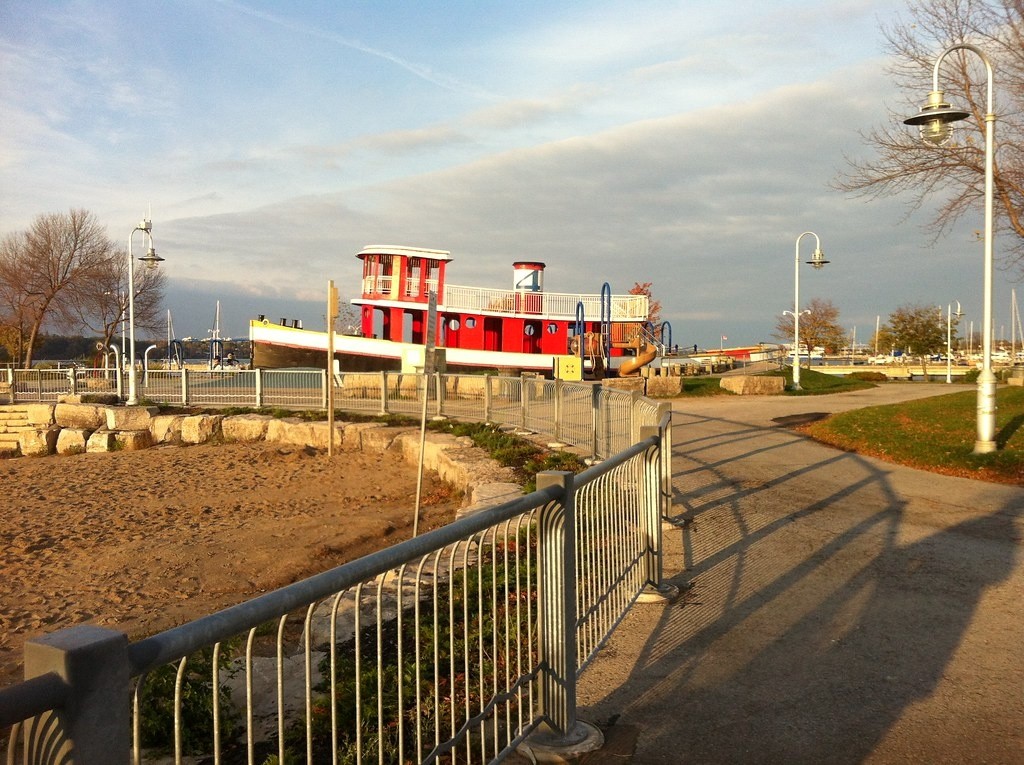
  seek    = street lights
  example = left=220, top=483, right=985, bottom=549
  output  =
left=900, top=42, right=1000, bottom=453
left=783, top=231, right=830, bottom=393
left=944, top=298, right=963, bottom=383
left=125, top=221, right=164, bottom=403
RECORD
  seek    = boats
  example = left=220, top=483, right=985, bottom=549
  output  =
left=247, top=241, right=781, bottom=370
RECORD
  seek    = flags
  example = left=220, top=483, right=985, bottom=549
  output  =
left=722, top=335, right=728, bottom=340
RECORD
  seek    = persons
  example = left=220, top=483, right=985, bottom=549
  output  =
left=206, top=351, right=241, bottom=372
left=692, top=344, right=697, bottom=354
left=674, top=343, right=678, bottom=352
